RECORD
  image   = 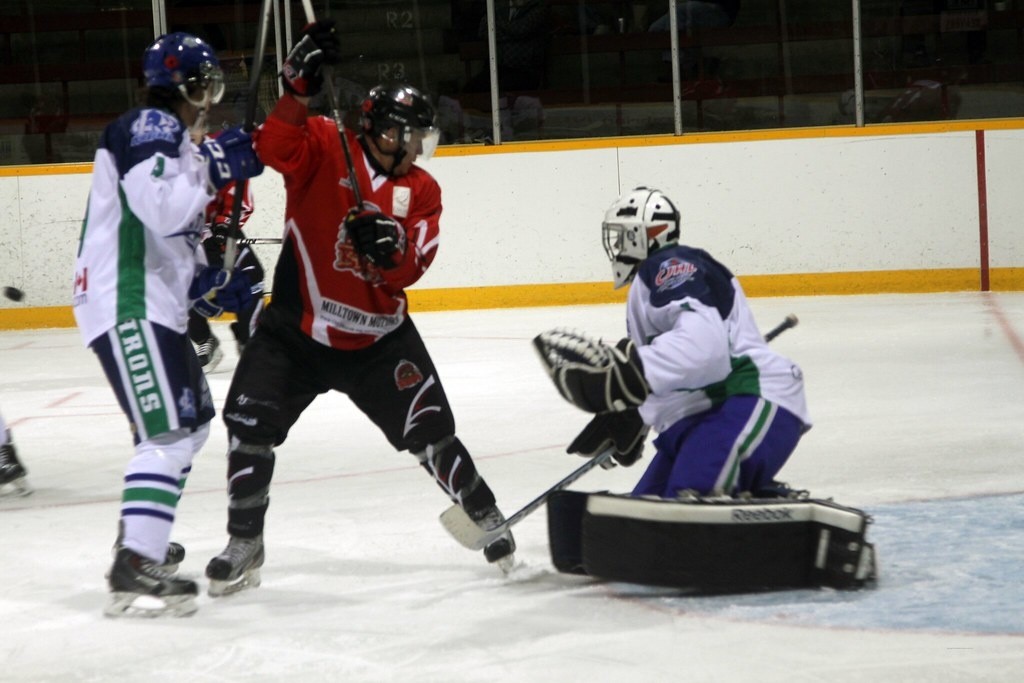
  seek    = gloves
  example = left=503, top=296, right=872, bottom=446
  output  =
left=198, top=123, right=264, bottom=194
left=285, top=18, right=364, bottom=83
left=202, top=224, right=228, bottom=251
left=345, top=208, right=405, bottom=270
left=188, top=264, right=257, bottom=322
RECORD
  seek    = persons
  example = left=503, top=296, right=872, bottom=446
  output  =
left=73, top=32, right=518, bottom=619
left=533, top=185, right=812, bottom=500
left=0, top=411, right=34, bottom=500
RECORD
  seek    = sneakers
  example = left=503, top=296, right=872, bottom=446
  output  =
left=194, top=332, right=223, bottom=373
left=205, top=536, right=265, bottom=597
left=472, top=504, right=516, bottom=576
left=0, top=428, right=35, bottom=497
left=114, top=520, right=185, bottom=575
left=103, top=548, right=201, bottom=618
left=230, top=321, right=250, bottom=355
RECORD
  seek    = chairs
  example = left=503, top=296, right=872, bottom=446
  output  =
left=0, top=0, right=1024, bottom=166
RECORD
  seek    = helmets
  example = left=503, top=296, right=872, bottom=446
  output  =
left=357, top=80, right=438, bottom=137
left=142, top=31, right=219, bottom=85
left=601, top=186, right=681, bottom=289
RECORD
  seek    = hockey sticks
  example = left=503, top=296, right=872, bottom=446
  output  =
left=224, top=0, right=274, bottom=271
left=439, top=312, right=801, bottom=553
left=301, top=0, right=385, bottom=263
left=214, top=234, right=285, bottom=246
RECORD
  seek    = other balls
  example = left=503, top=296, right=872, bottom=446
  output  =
left=2, top=286, right=24, bottom=303
